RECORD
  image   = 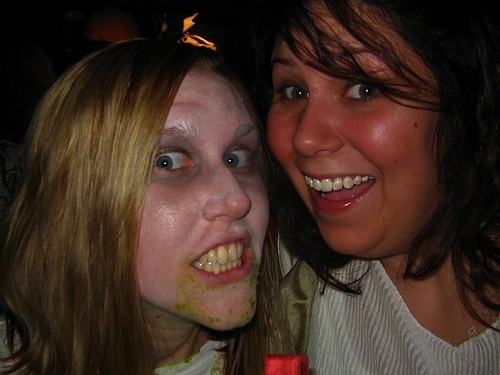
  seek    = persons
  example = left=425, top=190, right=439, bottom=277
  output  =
left=2, top=36, right=302, bottom=375
left=254, top=0, right=499, bottom=375
